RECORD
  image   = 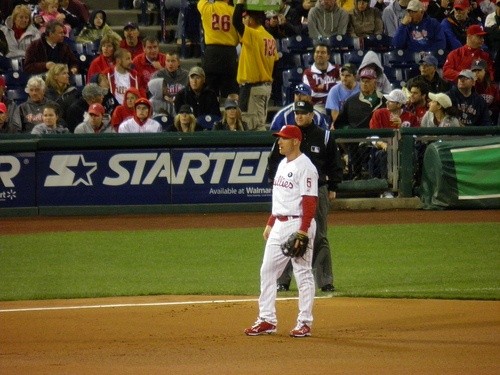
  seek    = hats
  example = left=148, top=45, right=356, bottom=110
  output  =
left=180, top=105, right=193, bottom=114
left=467, top=24, right=486, bottom=35
left=123, top=21, right=139, bottom=32
left=88, top=102, right=104, bottom=117
left=293, top=99, right=313, bottom=113
left=135, top=100, right=151, bottom=109
left=341, top=63, right=357, bottom=76
left=224, top=100, right=239, bottom=110
left=418, top=55, right=438, bottom=68
left=428, top=92, right=453, bottom=108
left=453, top=0, right=470, bottom=11
left=471, top=60, right=485, bottom=71
left=359, top=67, right=377, bottom=79
left=0, top=101, right=7, bottom=113
left=272, top=124, right=302, bottom=142
left=383, top=89, right=406, bottom=104
left=188, top=67, right=206, bottom=84
left=456, top=69, right=475, bottom=80
left=406, top=0, right=420, bottom=12
left=295, top=83, right=312, bottom=96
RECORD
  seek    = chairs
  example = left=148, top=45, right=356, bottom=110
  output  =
left=276, top=34, right=419, bottom=105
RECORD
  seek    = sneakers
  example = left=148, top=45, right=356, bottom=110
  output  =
left=290, top=322, right=311, bottom=336
left=245, top=319, right=276, bottom=335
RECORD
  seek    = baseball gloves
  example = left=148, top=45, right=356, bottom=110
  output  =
left=279, top=230, right=309, bottom=258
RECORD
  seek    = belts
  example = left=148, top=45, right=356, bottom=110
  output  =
left=277, top=216, right=299, bottom=221
left=253, top=82, right=270, bottom=87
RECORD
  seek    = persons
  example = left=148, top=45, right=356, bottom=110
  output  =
left=266, top=99, right=335, bottom=292
left=244, top=125, right=319, bottom=338
left=0, top=0, right=500, bottom=198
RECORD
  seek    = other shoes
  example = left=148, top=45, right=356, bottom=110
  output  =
left=322, top=284, right=334, bottom=292
left=276, top=284, right=289, bottom=291
